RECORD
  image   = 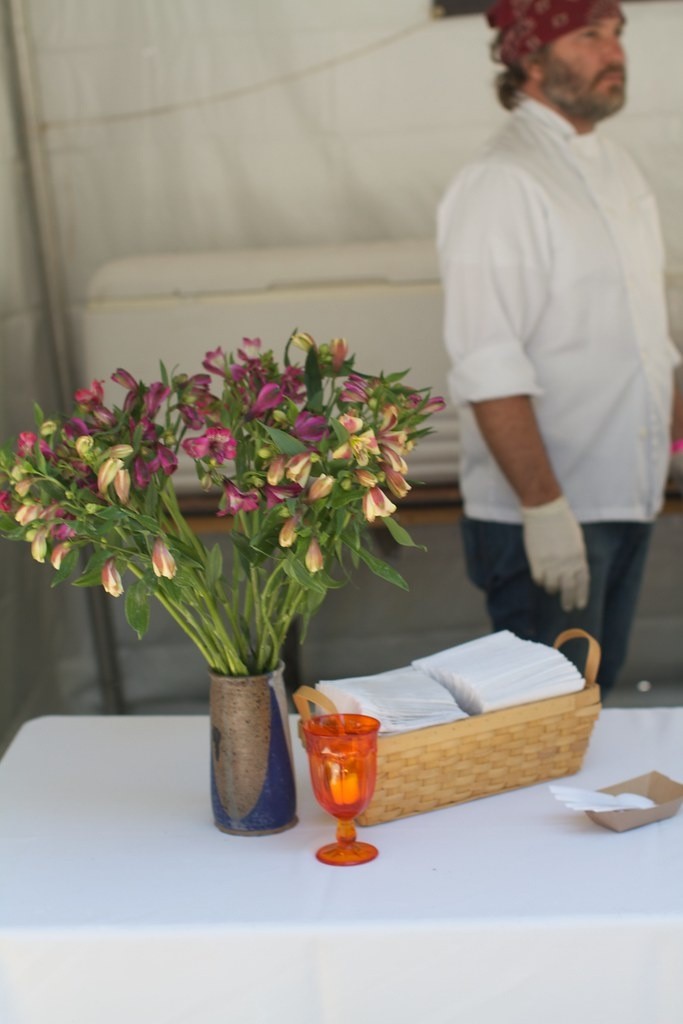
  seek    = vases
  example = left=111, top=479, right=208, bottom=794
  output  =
left=208, top=660, right=298, bottom=836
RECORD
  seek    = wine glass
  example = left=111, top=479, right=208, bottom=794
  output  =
left=300, top=713, right=378, bottom=866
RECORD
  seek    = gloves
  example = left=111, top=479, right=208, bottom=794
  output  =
left=519, top=495, right=590, bottom=612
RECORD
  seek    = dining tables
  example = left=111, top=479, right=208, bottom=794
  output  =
left=0, top=707, right=683, bottom=1024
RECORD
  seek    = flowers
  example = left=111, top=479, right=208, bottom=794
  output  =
left=0, top=327, right=447, bottom=675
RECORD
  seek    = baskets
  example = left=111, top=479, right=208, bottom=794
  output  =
left=293, top=627, right=603, bottom=833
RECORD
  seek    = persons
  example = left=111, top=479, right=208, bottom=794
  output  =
left=430, top=0, right=682, bottom=698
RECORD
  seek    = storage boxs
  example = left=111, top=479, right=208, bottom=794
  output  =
left=292, top=630, right=602, bottom=827
